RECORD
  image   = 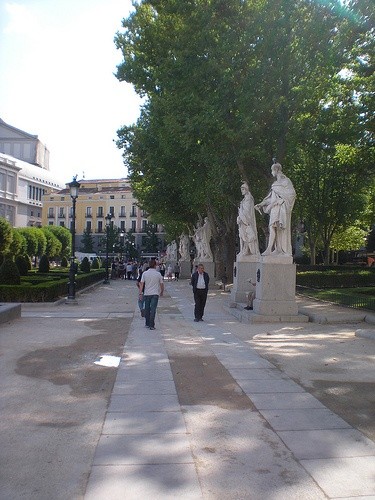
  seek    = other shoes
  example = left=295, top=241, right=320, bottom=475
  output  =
left=177, top=279, right=178, bottom=281
left=141, top=310, right=144, bottom=317
left=175, top=279, right=176, bottom=280
left=193, top=317, right=204, bottom=322
left=144, top=325, right=149, bottom=328
left=150, top=327, right=156, bottom=330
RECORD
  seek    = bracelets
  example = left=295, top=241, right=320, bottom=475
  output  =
left=139, top=293, right=143, bottom=295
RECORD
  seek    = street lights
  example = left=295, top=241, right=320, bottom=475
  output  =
left=103, top=214, right=112, bottom=284
left=119, top=230, right=135, bottom=280
left=65, top=177, right=81, bottom=304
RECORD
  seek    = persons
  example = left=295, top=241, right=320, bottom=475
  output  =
left=191, top=263, right=209, bottom=322
left=139, top=260, right=164, bottom=330
left=253, top=158, right=297, bottom=256
left=136, top=266, right=149, bottom=317
left=244, top=277, right=256, bottom=310
left=179, top=231, right=190, bottom=258
left=192, top=217, right=213, bottom=262
left=236, top=181, right=260, bottom=256
left=111, top=257, right=166, bottom=280
left=167, top=264, right=173, bottom=282
left=166, top=240, right=177, bottom=260
left=174, top=262, right=181, bottom=282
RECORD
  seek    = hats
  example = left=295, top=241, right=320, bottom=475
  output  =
left=127, top=263, right=131, bottom=265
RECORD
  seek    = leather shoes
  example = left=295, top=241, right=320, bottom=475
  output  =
left=244, top=306, right=253, bottom=310
